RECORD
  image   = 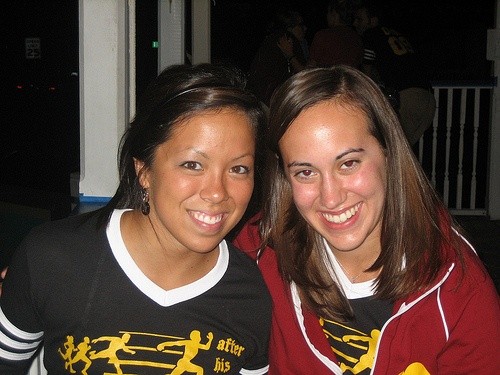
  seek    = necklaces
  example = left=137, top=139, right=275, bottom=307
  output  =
left=334, top=255, right=379, bottom=284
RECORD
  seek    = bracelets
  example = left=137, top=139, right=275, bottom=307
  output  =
left=288, top=56, right=295, bottom=73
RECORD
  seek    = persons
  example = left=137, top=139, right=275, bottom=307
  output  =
left=254, top=0, right=436, bottom=148
left=0, top=65, right=500, bottom=375
left=0, top=62, right=275, bottom=375
